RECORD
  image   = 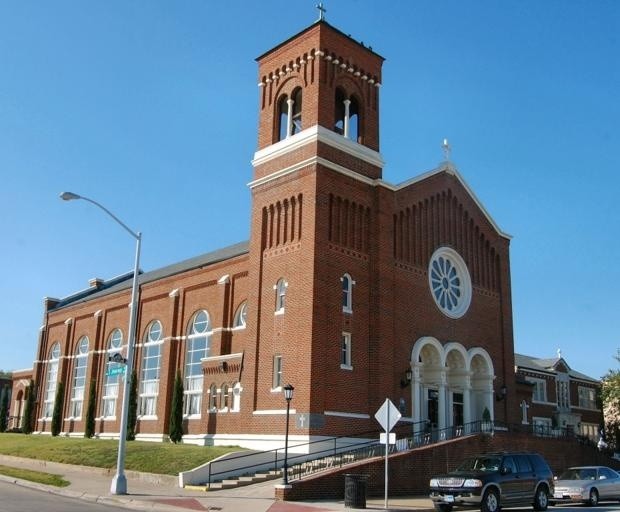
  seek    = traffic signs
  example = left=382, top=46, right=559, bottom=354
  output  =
left=107, top=366, right=125, bottom=375
left=109, top=356, right=126, bottom=363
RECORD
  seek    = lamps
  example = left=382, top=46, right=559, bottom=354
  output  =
left=399, top=365, right=413, bottom=390
left=495, top=385, right=508, bottom=403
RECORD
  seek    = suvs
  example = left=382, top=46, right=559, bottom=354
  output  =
left=428, top=452, right=556, bottom=511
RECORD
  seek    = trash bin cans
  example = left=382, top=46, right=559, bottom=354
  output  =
left=344, top=474, right=368, bottom=508
left=425, top=422, right=439, bottom=442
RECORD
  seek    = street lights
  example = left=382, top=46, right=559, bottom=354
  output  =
left=282, top=381, right=294, bottom=484
left=58, top=190, right=144, bottom=494
left=597, top=436, right=608, bottom=466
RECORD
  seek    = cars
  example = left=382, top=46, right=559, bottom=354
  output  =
left=548, top=466, right=620, bottom=505
